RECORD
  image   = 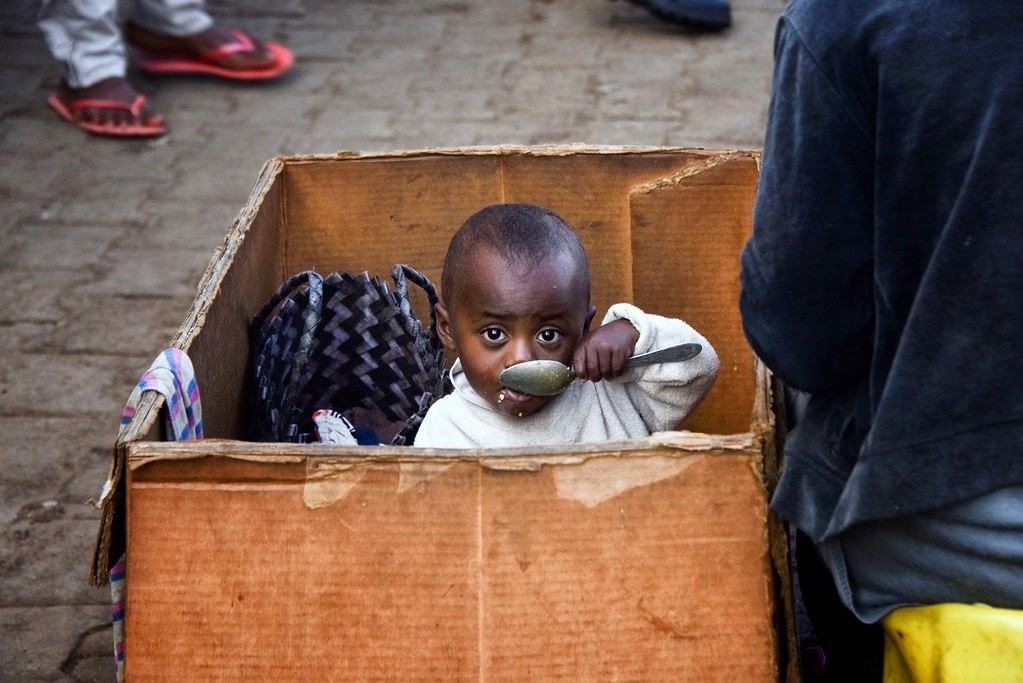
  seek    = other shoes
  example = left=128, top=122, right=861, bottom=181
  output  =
left=631, top=0, right=734, bottom=32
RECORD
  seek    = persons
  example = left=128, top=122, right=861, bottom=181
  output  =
left=411, top=202, right=720, bottom=450
left=741, top=1, right=1023, bottom=683
left=38, top=0, right=296, bottom=136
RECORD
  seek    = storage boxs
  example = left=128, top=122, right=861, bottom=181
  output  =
left=87, top=146, right=808, bottom=682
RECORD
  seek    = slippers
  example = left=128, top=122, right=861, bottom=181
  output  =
left=132, top=25, right=293, bottom=79
left=46, top=91, right=167, bottom=135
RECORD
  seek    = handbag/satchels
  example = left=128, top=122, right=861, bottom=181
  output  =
left=240, top=262, right=445, bottom=445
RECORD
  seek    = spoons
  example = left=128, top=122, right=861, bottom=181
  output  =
left=499, top=343, right=702, bottom=397
